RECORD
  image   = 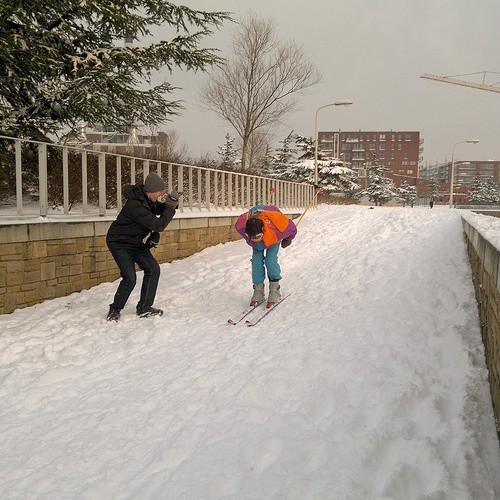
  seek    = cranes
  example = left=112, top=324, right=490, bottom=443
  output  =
left=420, top=70, right=500, bottom=92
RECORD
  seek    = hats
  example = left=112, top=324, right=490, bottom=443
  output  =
left=144, top=172, right=167, bottom=193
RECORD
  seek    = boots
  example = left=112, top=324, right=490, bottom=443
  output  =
left=268, top=281, right=281, bottom=303
left=251, top=281, right=266, bottom=304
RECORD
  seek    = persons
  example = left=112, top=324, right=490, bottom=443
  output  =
left=235, top=205, right=297, bottom=308
left=106, top=172, right=183, bottom=321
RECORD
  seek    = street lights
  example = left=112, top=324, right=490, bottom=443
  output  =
left=315, top=100, right=353, bottom=186
left=450, top=140, right=480, bottom=208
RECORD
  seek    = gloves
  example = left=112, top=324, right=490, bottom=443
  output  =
left=165, top=189, right=181, bottom=210
left=281, top=238, right=291, bottom=249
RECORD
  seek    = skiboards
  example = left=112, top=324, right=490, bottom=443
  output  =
left=228, top=292, right=292, bottom=326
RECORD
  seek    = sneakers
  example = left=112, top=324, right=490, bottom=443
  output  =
left=137, top=306, right=164, bottom=317
left=107, top=307, right=121, bottom=323
